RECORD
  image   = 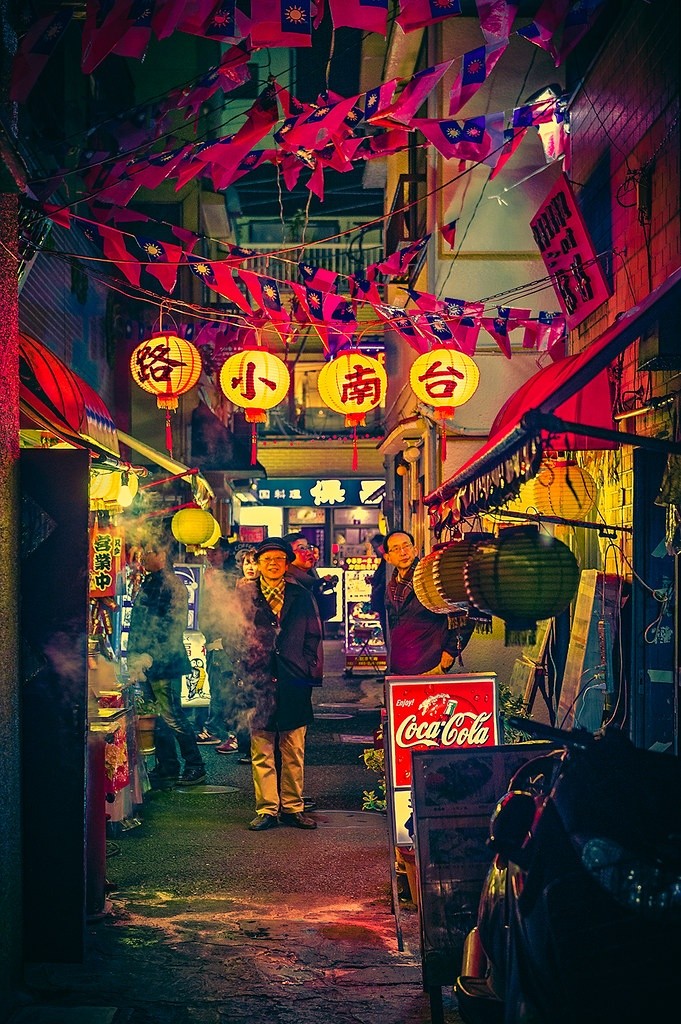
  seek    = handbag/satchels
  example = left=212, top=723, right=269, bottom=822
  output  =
left=318, top=583, right=337, bottom=620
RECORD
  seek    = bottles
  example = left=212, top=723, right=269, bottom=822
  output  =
left=427, top=699, right=457, bottom=750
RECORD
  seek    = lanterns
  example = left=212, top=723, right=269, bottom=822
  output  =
left=130, top=331, right=202, bottom=459
left=170, top=508, right=214, bottom=546
left=200, top=518, right=221, bottom=548
left=432, top=531, right=496, bottom=622
left=409, top=342, right=479, bottom=463
left=51, top=443, right=138, bottom=511
left=412, top=541, right=460, bottom=618
left=463, top=523, right=580, bottom=648
left=219, top=343, right=291, bottom=464
left=533, top=459, right=597, bottom=525
left=317, top=348, right=387, bottom=471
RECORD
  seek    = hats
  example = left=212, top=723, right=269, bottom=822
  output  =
left=254, top=537, right=296, bottom=562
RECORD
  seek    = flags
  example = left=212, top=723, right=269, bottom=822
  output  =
left=0, top=2, right=570, bottom=362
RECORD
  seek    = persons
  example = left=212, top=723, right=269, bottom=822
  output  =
left=364, top=533, right=391, bottom=683
left=186, top=535, right=261, bottom=761
left=127, top=535, right=208, bottom=786
left=276, top=533, right=321, bottom=814
left=383, top=530, right=479, bottom=676
left=238, top=537, right=324, bottom=830
left=306, top=544, right=337, bottom=593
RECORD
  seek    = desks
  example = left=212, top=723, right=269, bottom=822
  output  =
left=343, top=626, right=386, bottom=681
left=87, top=682, right=149, bottom=839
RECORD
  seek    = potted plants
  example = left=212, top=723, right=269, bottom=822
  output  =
left=130, top=694, right=161, bottom=750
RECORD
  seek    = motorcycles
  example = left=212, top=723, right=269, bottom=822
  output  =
left=455, top=716, right=681, bottom=1024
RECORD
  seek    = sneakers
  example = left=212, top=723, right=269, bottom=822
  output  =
left=146, top=765, right=179, bottom=778
left=177, top=767, right=207, bottom=786
left=213, top=735, right=240, bottom=754
left=277, top=812, right=317, bottom=828
left=248, top=813, right=276, bottom=831
left=194, top=731, right=221, bottom=744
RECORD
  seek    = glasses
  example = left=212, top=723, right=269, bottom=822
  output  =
left=300, top=543, right=314, bottom=553
left=260, top=557, right=284, bottom=563
left=389, top=544, right=412, bottom=552
left=141, top=548, right=157, bottom=556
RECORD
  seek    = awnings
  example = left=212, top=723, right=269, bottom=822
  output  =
left=422, top=266, right=680, bottom=542
left=15, top=330, right=121, bottom=466
left=190, top=399, right=268, bottom=480
left=116, top=428, right=215, bottom=524
